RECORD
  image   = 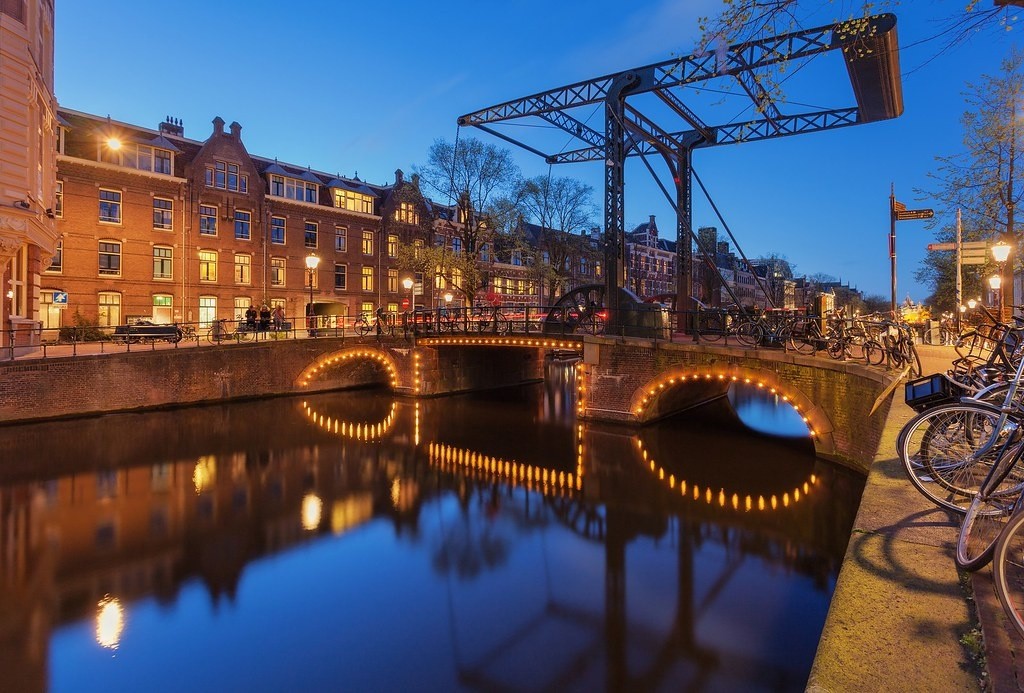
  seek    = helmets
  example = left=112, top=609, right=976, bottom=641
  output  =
left=262, top=304, right=267, bottom=309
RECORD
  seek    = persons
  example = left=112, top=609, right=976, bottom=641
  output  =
left=245, top=305, right=257, bottom=331
left=272, top=305, right=284, bottom=331
left=259, top=305, right=270, bottom=330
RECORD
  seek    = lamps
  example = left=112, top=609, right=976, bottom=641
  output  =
left=46, top=209, right=55, bottom=220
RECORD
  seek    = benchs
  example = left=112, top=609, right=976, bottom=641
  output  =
left=310, top=327, right=349, bottom=337
left=239, top=322, right=291, bottom=342
left=114, top=323, right=179, bottom=352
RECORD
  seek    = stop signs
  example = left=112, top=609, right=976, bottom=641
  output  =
left=402, top=299, right=410, bottom=308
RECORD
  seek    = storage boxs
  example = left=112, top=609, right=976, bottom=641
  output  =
left=905, top=373, right=970, bottom=424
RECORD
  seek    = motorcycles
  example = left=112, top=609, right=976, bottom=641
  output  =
left=122, top=311, right=182, bottom=344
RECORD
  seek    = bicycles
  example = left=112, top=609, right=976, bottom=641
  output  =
left=555, top=303, right=604, bottom=335
left=435, top=304, right=470, bottom=332
left=207, top=315, right=255, bottom=343
left=180, top=326, right=197, bottom=342
left=353, top=308, right=393, bottom=335
left=698, top=307, right=1023, bottom=634
left=471, top=302, right=510, bottom=333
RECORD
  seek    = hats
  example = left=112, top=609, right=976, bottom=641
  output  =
left=249, top=305, right=254, bottom=308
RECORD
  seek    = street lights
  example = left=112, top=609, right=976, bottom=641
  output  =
left=305, top=252, right=319, bottom=336
left=961, top=305, right=966, bottom=320
left=989, top=274, right=1001, bottom=306
left=403, top=277, right=414, bottom=332
left=968, top=299, right=976, bottom=322
left=991, top=241, right=1011, bottom=324
